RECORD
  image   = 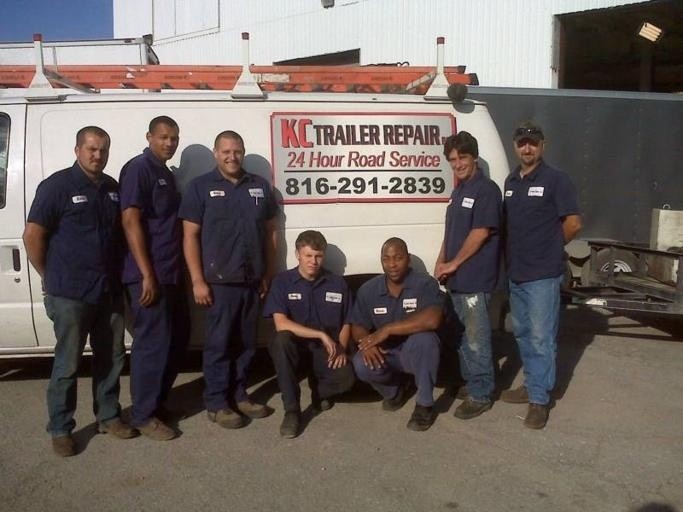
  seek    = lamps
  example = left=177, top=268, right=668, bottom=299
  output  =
left=634, top=18, right=665, bottom=45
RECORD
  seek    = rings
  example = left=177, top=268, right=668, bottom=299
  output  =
left=368, top=340, right=371, bottom=342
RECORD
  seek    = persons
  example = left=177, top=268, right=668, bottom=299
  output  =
left=350, top=237, right=445, bottom=431
left=22, top=126, right=140, bottom=457
left=263, top=229, right=356, bottom=438
left=177, top=130, right=280, bottom=429
left=501, top=121, right=581, bottom=429
left=118, top=116, right=192, bottom=440
left=434, top=131, right=502, bottom=419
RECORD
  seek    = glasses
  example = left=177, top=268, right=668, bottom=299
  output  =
left=517, top=126, right=535, bottom=135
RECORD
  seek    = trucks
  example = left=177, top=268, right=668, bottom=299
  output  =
left=0, top=32, right=514, bottom=374
left=455, top=82, right=683, bottom=341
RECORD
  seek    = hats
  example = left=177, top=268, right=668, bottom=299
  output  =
left=512, top=120, right=545, bottom=144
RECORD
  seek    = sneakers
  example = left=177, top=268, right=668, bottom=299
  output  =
left=406, top=402, right=440, bottom=432
left=206, top=407, right=246, bottom=428
left=231, top=397, right=269, bottom=418
left=52, top=428, right=77, bottom=457
left=451, top=381, right=549, bottom=430
left=313, top=395, right=333, bottom=410
left=128, top=412, right=178, bottom=442
left=382, top=377, right=416, bottom=413
left=97, top=416, right=140, bottom=440
left=279, top=410, right=302, bottom=439
